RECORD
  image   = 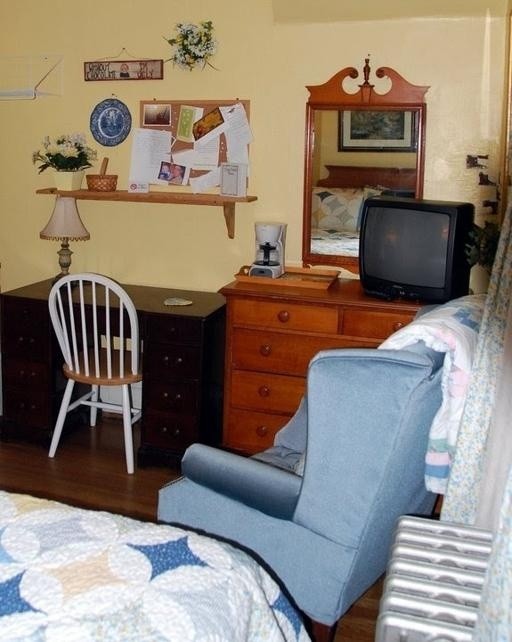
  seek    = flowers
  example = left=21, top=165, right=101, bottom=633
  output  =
left=162, top=19, right=219, bottom=76
left=30, top=132, right=102, bottom=172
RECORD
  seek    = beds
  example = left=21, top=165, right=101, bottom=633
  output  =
left=0, top=490, right=316, bottom=642
left=307, top=160, right=414, bottom=262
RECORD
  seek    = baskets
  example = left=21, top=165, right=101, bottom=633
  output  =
left=86, top=174, right=118, bottom=191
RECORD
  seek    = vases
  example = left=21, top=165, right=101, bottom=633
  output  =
left=51, top=169, right=86, bottom=193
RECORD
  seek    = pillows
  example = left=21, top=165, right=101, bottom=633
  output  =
left=312, top=185, right=387, bottom=239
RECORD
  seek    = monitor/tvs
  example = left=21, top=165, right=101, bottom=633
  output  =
left=359, top=196, right=474, bottom=304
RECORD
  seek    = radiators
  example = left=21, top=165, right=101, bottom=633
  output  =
left=374, top=508, right=494, bottom=640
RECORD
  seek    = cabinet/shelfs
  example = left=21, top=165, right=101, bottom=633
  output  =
left=218, top=276, right=435, bottom=457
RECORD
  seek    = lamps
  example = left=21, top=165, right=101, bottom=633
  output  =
left=39, top=197, right=93, bottom=286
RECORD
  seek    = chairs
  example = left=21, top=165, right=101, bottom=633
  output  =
left=154, top=335, right=452, bottom=631
left=48, top=273, right=144, bottom=473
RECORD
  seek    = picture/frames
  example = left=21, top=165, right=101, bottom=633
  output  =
left=82, top=58, right=165, bottom=83
left=334, top=107, right=419, bottom=154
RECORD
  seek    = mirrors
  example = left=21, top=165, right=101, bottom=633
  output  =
left=301, top=57, right=431, bottom=271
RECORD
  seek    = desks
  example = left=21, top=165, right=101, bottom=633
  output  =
left=2, top=276, right=232, bottom=470
